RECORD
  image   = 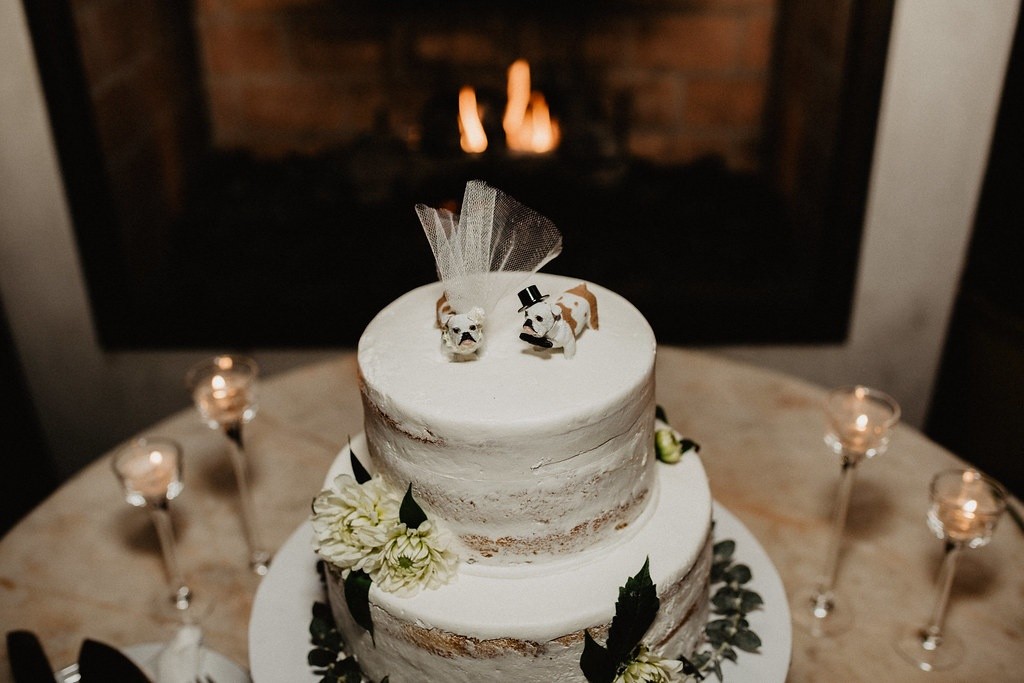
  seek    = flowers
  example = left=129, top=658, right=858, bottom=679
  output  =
left=579, top=555, right=689, bottom=683
left=655, top=428, right=701, bottom=464
left=308, top=433, right=461, bottom=647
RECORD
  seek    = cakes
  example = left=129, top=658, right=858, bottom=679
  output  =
left=311, top=270, right=712, bottom=683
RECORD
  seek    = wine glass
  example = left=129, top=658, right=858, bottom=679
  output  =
left=183, top=353, right=280, bottom=580
left=889, top=468, right=1012, bottom=673
left=791, top=383, right=902, bottom=639
left=110, top=435, right=217, bottom=625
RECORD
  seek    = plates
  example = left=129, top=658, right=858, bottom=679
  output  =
left=53, top=639, right=254, bottom=683
left=246, top=495, right=794, bottom=683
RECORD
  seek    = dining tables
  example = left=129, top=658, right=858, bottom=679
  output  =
left=0, top=344, right=1024, bottom=683
